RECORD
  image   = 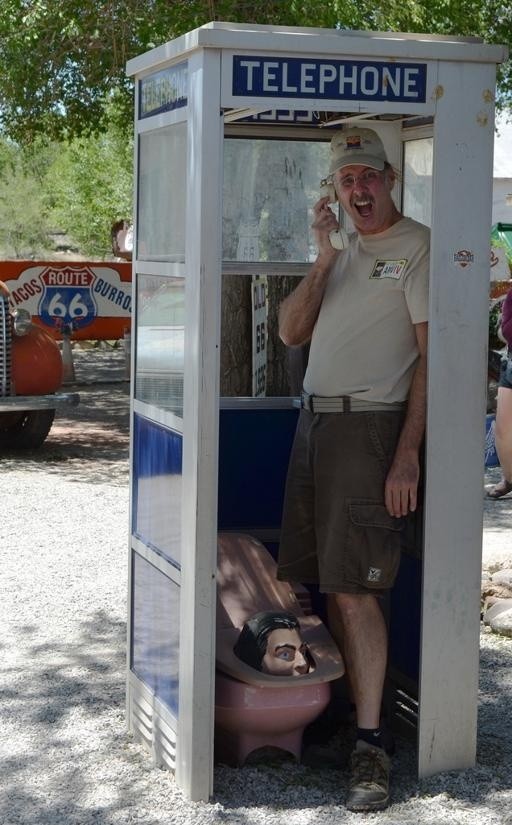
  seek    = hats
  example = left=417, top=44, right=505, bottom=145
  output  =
left=329, top=126, right=387, bottom=173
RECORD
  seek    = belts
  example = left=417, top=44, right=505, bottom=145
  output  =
left=298, top=389, right=405, bottom=415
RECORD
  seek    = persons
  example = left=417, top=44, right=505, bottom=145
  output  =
left=270, top=124, right=434, bottom=814
left=485, top=284, right=512, bottom=501
left=233, top=611, right=317, bottom=678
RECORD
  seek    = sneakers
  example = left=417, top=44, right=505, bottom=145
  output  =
left=346, top=741, right=392, bottom=813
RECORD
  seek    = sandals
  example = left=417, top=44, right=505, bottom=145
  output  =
left=487, top=480, right=512, bottom=498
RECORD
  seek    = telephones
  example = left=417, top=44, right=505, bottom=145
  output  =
left=318, top=184, right=349, bottom=250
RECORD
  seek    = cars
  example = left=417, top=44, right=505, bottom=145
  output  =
left=1, top=280, right=81, bottom=451
